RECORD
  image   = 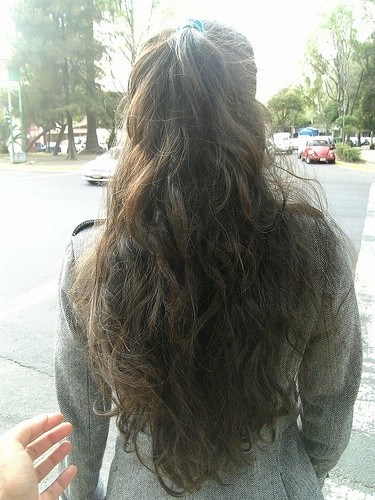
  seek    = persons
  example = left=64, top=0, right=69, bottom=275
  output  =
left=0, top=412, right=76, bottom=499
left=53, top=21, right=362, bottom=500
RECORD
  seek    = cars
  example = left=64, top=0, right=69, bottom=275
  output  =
left=82, top=146, right=121, bottom=185
left=299, top=139, right=335, bottom=164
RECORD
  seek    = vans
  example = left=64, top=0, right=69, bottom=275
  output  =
left=271, top=133, right=292, bottom=154
